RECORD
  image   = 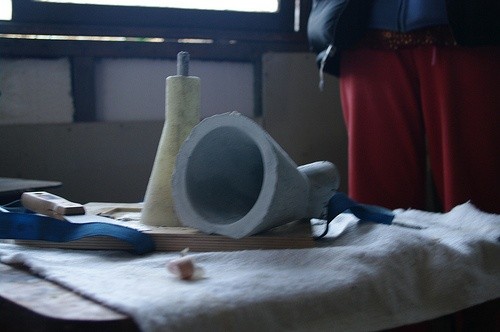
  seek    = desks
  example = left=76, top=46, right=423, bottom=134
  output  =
left=0, top=200, right=500, bottom=331
